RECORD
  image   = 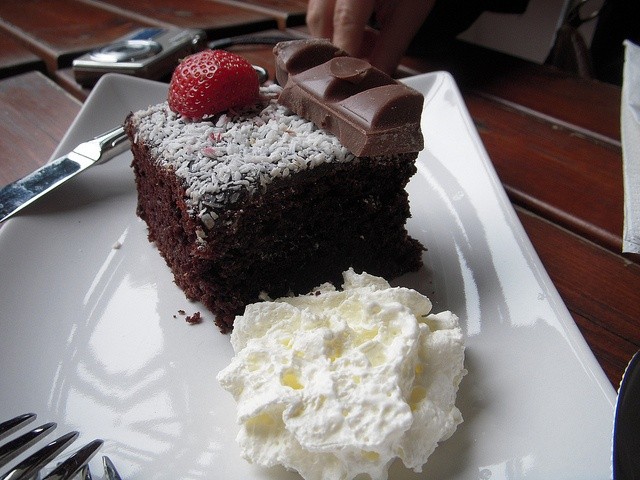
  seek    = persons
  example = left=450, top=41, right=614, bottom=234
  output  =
left=306, top=0, right=432, bottom=75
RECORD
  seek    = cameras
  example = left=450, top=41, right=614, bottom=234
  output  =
left=72, top=27, right=207, bottom=89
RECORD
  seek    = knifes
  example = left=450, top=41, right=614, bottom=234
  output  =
left=1, top=66, right=269, bottom=222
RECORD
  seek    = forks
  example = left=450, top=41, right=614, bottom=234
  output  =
left=57, top=457, right=121, bottom=479
left=1, top=413, right=103, bottom=479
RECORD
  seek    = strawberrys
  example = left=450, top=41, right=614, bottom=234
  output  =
left=166, top=49, right=260, bottom=119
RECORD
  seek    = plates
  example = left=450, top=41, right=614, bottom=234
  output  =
left=0, top=69, right=620, bottom=479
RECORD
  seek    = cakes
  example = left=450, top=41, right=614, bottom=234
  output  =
left=123, top=85, right=429, bottom=334
